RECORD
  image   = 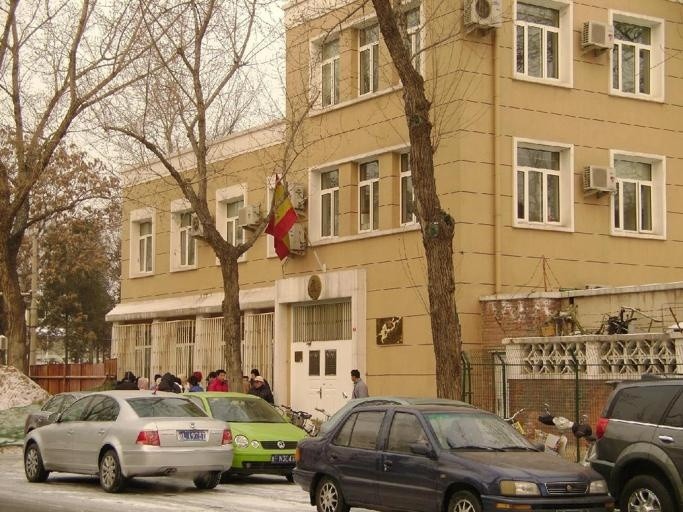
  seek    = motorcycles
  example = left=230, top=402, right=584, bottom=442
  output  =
left=498, top=405, right=597, bottom=468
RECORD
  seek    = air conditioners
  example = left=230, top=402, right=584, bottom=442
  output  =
left=580, top=163, right=618, bottom=196
left=461, top=0, right=509, bottom=31
left=189, top=208, right=208, bottom=240
left=579, top=18, right=619, bottom=54
left=285, top=183, right=307, bottom=213
left=235, top=204, right=263, bottom=228
left=283, top=222, right=308, bottom=254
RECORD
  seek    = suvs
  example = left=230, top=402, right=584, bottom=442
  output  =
left=588, top=379, right=683, bottom=511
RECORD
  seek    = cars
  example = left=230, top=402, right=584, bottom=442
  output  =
left=22, top=388, right=235, bottom=492
left=24, top=390, right=92, bottom=435
left=178, top=390, right=309, bottom=483
left=292, top=403, right=616, bottom=511
left=306, top=395, right=477, bottom=440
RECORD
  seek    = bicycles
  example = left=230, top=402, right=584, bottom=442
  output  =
left=271, top=401, right=330, bottom=438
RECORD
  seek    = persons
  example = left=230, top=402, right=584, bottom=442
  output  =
left=106, top=370, right=274, bottom=421
left=351, top=370, right=368, bottom=400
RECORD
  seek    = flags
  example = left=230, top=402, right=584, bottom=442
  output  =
left=264, top=175, right=297, bottom=261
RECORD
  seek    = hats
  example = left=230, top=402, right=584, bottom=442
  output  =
left=188, top=371, right=201, bottom=386
left=255, top=376, right=264, bottom=382
